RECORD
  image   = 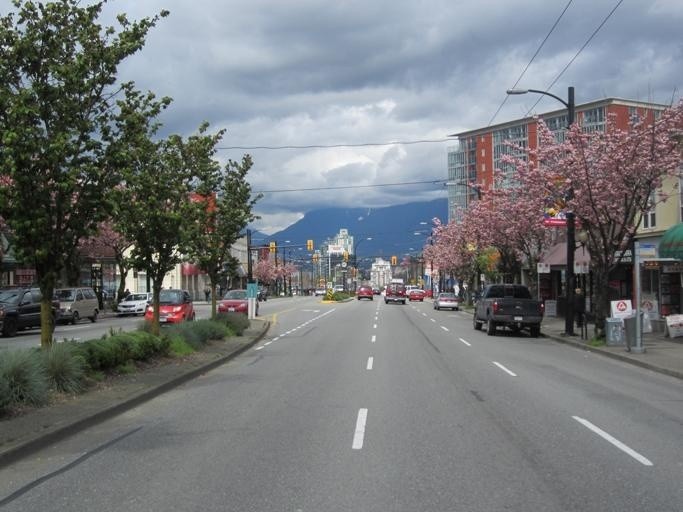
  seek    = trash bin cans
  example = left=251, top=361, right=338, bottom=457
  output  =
left=623, top=312, right=643, bottom=352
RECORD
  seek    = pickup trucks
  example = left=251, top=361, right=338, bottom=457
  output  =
left=470, top=281, right=546, bottom=339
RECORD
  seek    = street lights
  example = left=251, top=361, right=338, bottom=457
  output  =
left=400, top=217, right=437, bottom=296
left=274, top=236, right=314, bottom=297
left=442, top=180, right=485, bottom=299
left=501, top=86, right=580, bottom=338
left=353, top=236, right=373, bottom=292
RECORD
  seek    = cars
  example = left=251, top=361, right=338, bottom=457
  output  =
left=143, top=287, right=195, bottom=328
left=431, top=291, right=461, bottom=311
left=218, top=289, right=259, bottom=319
left=115, top=292, right=153, bottom=317
left=313, top=281, right=425, bottom=305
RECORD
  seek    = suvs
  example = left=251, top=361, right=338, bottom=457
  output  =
left=257, top=286, right=268, bottom=302
left=0, top=284, right=63, bottom=338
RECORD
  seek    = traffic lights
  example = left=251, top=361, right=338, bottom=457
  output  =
left=392, top=257, right=397, bottom=265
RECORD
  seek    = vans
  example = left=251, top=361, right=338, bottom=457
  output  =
left=54, top=286, right=101, bottom=327
